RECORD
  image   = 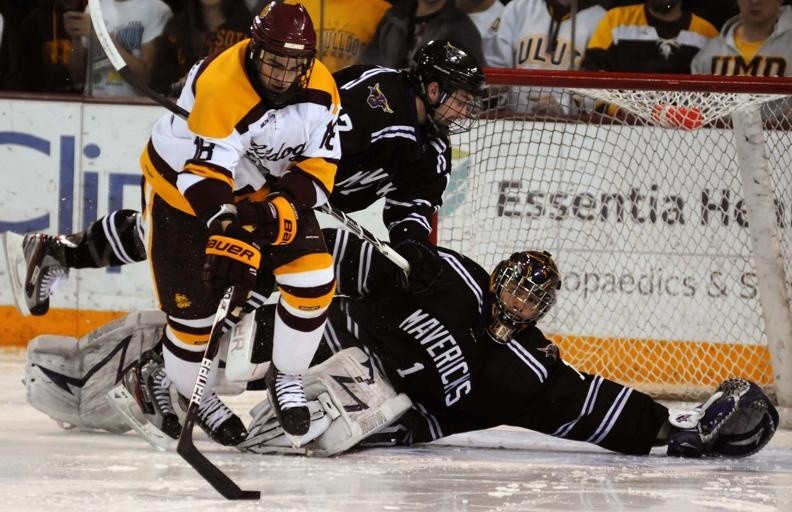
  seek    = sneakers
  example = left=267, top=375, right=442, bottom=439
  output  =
left=22, top=233, right=70, bottom=316
left=178, top=390, right=250, bottom=448
left=263, top=362, right=313, bottom=437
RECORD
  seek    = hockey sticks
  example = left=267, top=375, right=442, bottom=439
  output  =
left=89, top=0, right=409, bottom=273
left=176, top=287, right=261, bottom=501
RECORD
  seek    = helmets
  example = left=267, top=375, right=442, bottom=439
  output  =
left=406, top=41, right=484, bottom=137
left=248, top=2, right=318, bottom=101
left=484, top=252, right=562, bottom=344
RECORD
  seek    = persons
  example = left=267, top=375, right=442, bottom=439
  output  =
left=20, top=34, right=483, bottom=314
left=67, top=1, right=792, bottom=128
left=140, top=0, right=343, bottom=444
left=21, top=243, right=780, bottom=460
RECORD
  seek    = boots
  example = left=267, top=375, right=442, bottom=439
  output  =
left=124, top=347, right=185, bottom=441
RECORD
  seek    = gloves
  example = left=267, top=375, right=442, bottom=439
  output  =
left=242, top=195, right=300, bottom=250
left=208, top=231, right=262, bottom=310
